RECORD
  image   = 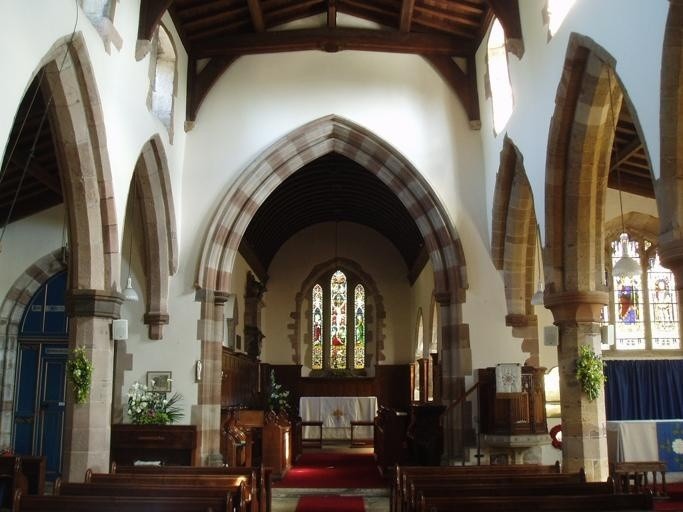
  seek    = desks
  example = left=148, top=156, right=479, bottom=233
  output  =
left=614, top=462, right=668, bottom=496
left=350, top=421, right=375, bottom=448
left=606, top=419, right=683, bottom=485
left=110, top=424, right=201, bottom=468
left=301, top=421, right=323, bottom=450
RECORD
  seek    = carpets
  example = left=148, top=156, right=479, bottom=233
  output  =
left=271, top=452, right=392, bottom=488
left=294, top=494, right=367, bottom=512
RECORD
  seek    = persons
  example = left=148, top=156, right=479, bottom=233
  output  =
left=650, top=274, right=676, bottom=332
left=615, top=275, right=640, bottom=326
left=331, top=293, right=345, bottom=345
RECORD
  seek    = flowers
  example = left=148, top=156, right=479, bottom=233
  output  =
left=566, top=343, right=608, bottom=401
left=264, top=368, right=297, bottom=420
left=127, top=380, right=186, bottom=425
left=63, top=342, right=96, bottom=405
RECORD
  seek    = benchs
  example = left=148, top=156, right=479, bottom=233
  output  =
left=390, top=461, right=652, bottom=512
left=11, top=461, right=273, bottom=512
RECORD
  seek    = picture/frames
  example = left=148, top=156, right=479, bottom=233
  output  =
left=146, top=371, right=172, bottom=393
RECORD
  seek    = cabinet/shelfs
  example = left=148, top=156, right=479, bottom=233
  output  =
left=477, top=365, right=548, bottom=436
left=262, top=422, right=292, bottom=482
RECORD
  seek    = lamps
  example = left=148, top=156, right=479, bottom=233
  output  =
left=530, top=218, right=544, bottom=305
left=607, top=65, right=644, bottom=275
left=122, top=171, right=140, bottom=302
